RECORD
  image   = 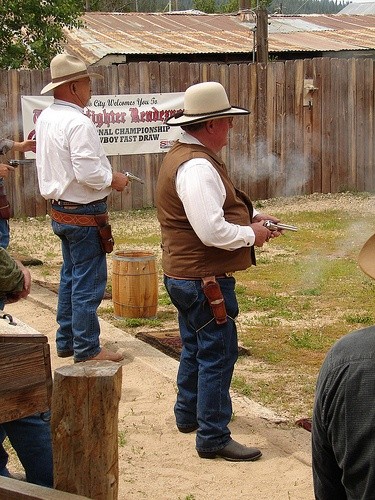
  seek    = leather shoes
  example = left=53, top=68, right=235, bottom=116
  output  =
left=197, top=439, right=262, bottom=461
left=179, top=423, right=199, bottom=433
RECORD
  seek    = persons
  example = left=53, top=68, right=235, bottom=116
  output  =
left=0, top=247, right=53, bottom=489
left=311, top=233, right=375, bottom=500
left=0, top=137, right=37, bottom=309
left=157, top=82, right=284, bottom=461
left=35, top=55, right=130, bottom=362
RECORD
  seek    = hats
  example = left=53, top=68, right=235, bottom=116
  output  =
left=40, top=55, right=105, bottom=95
left=357, top=233, right=375, bottom=281
left=166, top=81, right=250, bottom=127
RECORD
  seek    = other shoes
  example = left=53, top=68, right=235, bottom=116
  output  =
left=57, top=351, right=72, bottom=358
left=74, top=352, right=125, bottom=363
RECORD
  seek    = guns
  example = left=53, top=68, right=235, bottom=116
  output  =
left=9, top=160, right=33, bottom=168
left=263, top=219, right=298, bottom=243
left=125, top=171, right=144, bottom=184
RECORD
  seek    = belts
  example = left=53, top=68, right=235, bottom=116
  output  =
left=52, top=196, right=107, bottom=207
left=50, top=208, right=106, bottom=226
left=164, top=270, right=232, bottom=280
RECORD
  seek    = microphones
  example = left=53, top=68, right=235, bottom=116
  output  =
left=76, top=94, right=90, bottom=111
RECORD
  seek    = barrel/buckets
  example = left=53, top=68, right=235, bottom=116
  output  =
left=111, top=250, right=160, bottom=319
